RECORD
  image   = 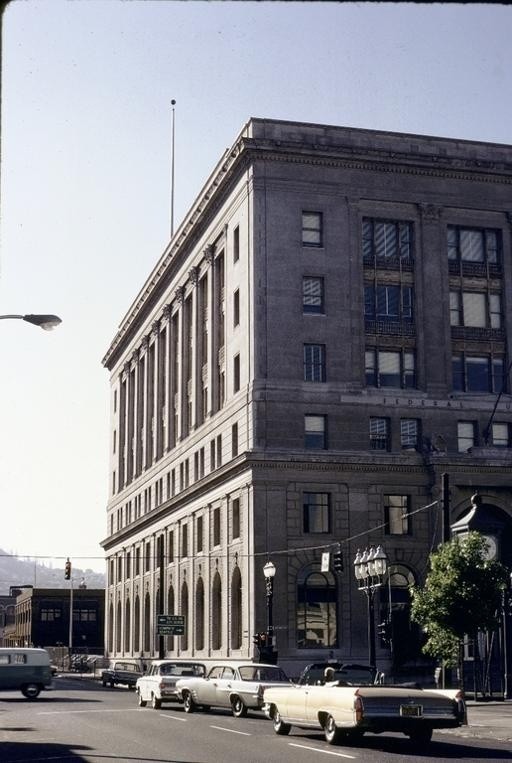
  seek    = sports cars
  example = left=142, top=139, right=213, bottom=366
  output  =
left=262, top=662, right=469, bottom=746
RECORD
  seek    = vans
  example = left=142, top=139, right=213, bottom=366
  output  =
left=54, top=654, right=105, bottom=673
left=0, top=648, right=53, bottom=699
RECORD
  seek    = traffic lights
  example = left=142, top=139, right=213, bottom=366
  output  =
left=64, top=562, right=70, bottom=580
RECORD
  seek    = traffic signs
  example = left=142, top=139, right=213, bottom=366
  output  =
left=352, top=543, right=387, bottom=683
left=157, top=614, right=185, bottom=637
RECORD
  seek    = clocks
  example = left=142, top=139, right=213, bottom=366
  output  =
left=478, top=533, right=500, bottom=562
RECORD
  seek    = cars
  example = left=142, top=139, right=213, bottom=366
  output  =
left=175, top=662, right=289, bottom=717
left=136, top=659, right=210, bottom=711
left=101, top=662, right=143, bottom=688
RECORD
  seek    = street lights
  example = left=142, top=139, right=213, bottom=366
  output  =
left=263, top=562, right=276, bottom=662
